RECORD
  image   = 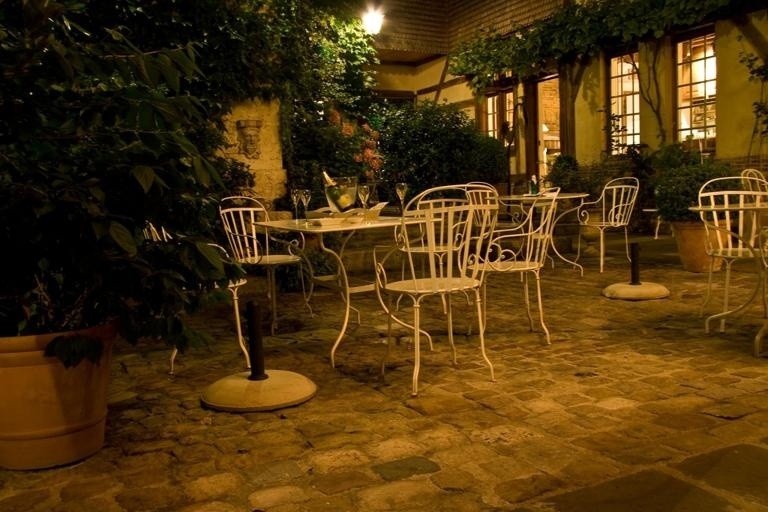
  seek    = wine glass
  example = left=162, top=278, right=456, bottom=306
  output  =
left=395, top=183, right=410, bottom=217
left=289, top=189, right=312, bottom=223
left=359, top=186, right=371, bottom=214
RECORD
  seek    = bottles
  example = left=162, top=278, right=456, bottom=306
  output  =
left=529, top=174, right=548, bottom=194
left=322, top=171, right=353, bottom=211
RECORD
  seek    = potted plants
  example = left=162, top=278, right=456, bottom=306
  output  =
left=0, top=0, right=246, bottom=474
left=655, top=156, right=745, bottom=273
left=464, top=135, right=510, bottom=215
